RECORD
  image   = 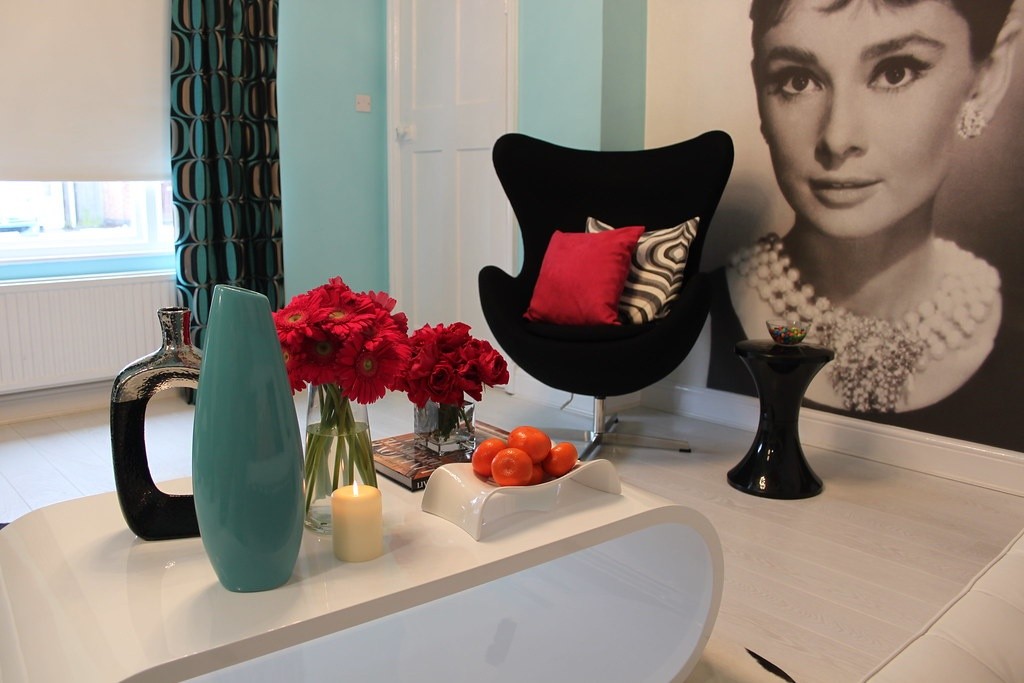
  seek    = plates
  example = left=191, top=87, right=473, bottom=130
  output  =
left=420, top=439, right=621, bottom=540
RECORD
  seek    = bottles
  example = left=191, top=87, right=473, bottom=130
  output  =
left=193, top=284, right=308, bottom=593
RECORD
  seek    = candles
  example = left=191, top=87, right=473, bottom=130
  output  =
left=330, top=477, right=385, bottom=564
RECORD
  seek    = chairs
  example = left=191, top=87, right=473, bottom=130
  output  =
left=479, top=130, right=735, bottom=461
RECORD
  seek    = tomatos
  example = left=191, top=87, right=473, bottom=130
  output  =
left=472, top=426, right=578, bottom=487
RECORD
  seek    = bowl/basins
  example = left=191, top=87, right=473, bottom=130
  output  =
left=765, top=318, right=811, bottom=345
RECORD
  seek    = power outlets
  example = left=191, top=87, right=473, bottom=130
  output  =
left=355, top=94, right=371, bottom=114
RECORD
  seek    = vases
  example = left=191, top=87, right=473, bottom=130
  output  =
left=191, top=284, right=307, bottom=594
left=110, top=306, right=204, bottom=542
left=412, top=397, right=476, bottom=456
left=302, top=380, right=378, bottom=534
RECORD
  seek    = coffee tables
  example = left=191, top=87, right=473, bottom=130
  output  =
left=0, top=420, right=725, bottom=682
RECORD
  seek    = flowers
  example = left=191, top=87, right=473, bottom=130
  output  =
left=270, top=274, right=411, bottom=516
left=389, top=321, right=510, bottom=440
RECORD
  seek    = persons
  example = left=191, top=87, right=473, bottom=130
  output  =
left=702, top=0, right=1024, bottom=451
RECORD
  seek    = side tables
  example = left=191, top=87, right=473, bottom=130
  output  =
left=727, top=338, right=835, bottom=499
